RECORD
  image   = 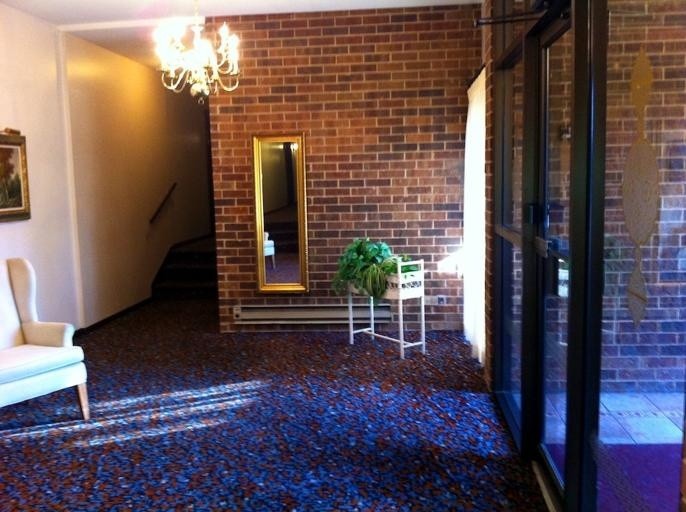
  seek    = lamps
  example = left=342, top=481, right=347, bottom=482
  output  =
left=151, top=0, right=241, bottom=104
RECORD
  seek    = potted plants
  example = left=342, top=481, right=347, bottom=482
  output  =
left=332, top=238, right=425, bottom=360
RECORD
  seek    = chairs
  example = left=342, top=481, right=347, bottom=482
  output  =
left=0, top=258, right=91, bottom=421
left=264, top=232, right=276, bottom=269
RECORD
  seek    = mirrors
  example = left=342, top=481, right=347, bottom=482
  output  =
left=252, top=131, right=309, bottom=295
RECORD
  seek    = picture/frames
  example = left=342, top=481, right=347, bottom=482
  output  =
left=0, top=135, right=31, bottom=223
